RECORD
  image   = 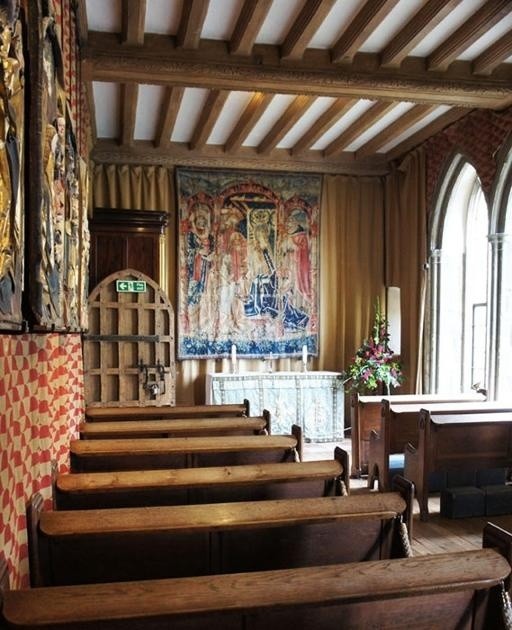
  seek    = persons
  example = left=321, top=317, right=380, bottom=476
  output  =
left=184, top=204, right=313, bottom=339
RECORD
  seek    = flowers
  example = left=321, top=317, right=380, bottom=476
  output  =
left=336, top=293, right=406, bottom=394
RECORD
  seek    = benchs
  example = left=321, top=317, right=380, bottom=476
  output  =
left=366, top=399, right=512, bottom=493
left=404, top=408, right=512, bottom=523
left=69, top=424, right=304, bottom=474
left=482, top=521, right=512, bottom=593
left=52, top=446, right=351, bottom=511
left=83, top=399, right=251, bottom=424
left=27, top=471, right=414, bottom=587
left=77, top=409, right=273, bottom=441
left=0, top=549, right=512, bottom=629
left=351, top=389, right=488, bottom=479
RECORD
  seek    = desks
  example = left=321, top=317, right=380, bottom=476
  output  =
left=205, top=373, right=346, bottom=442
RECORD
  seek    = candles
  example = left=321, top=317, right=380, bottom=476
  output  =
left=230, top=343, right=238, bottom=364
left=301, top=345, right=308, bottom=362
